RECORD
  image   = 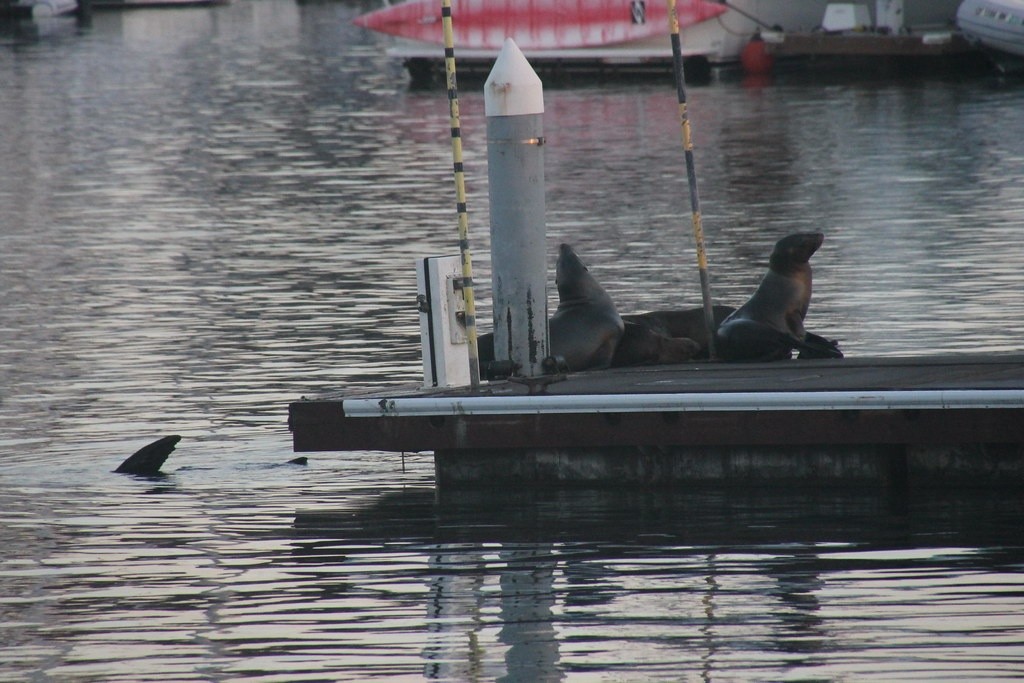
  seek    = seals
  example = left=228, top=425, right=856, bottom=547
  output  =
left=475, top=233, right=844, bottom=382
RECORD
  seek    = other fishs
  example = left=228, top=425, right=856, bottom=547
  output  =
left=112, top=433, right=309, bottom=479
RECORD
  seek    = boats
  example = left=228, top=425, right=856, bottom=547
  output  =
left=283, top=1, right=1023, bottom=531
